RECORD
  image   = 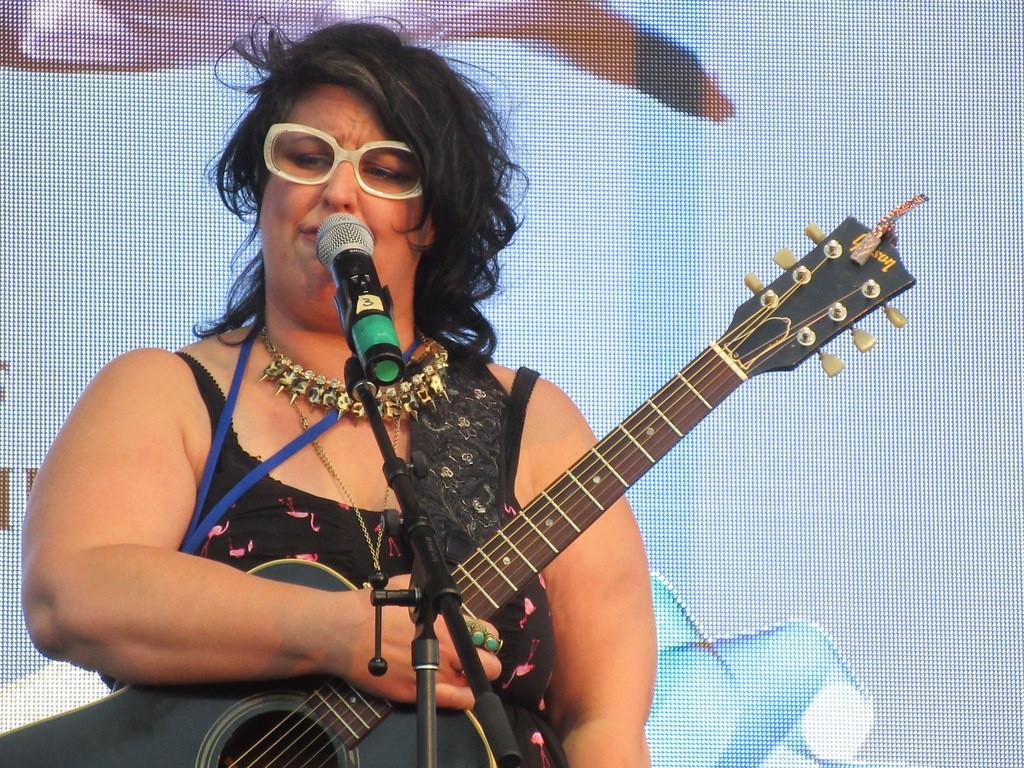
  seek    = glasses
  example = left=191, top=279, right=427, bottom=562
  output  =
left=263, top=121, right=425, bottom=200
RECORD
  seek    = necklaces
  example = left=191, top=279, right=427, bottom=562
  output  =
left=179, top=317, right=450, bottom=589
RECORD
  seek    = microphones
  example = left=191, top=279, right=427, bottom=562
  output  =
left=316, top=212, right=405, bottom=387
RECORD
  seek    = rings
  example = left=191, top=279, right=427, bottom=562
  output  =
left=466, top=619, right=504, bottom=655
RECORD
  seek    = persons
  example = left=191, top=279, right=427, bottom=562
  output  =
left=20, top=15, right=657, bottom=768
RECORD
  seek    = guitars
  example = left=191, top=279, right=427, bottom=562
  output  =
left=0, top=193, right=930, bottom=768
